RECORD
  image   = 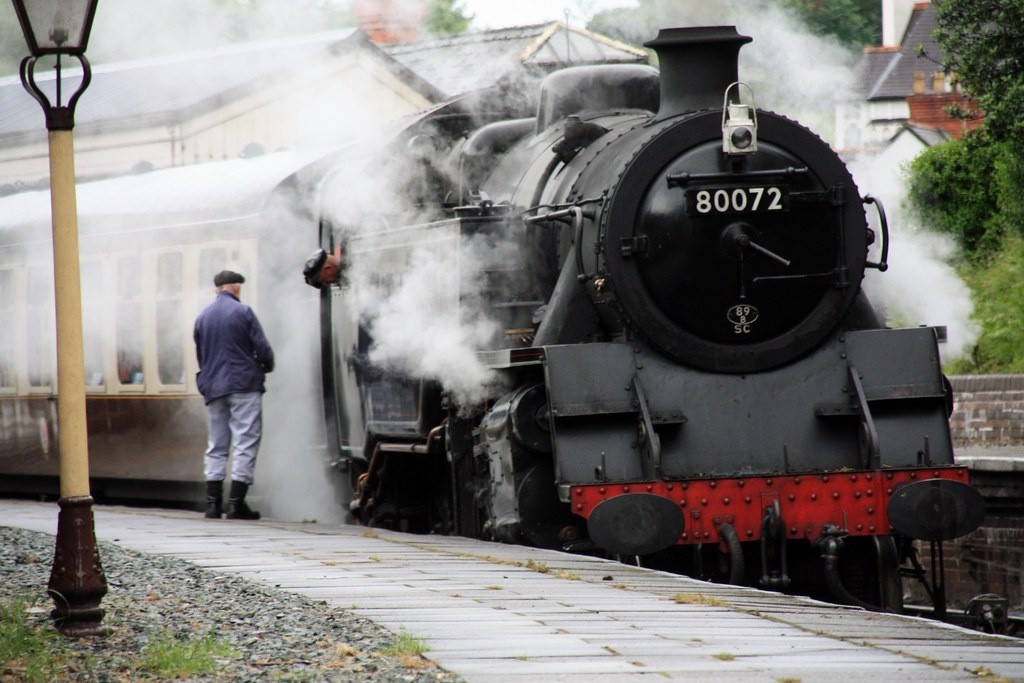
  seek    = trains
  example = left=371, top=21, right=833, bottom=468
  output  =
left=0, top=26, right=1024, bottom=635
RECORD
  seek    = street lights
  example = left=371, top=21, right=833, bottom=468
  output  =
left=12, top=0, right=110, bottom=628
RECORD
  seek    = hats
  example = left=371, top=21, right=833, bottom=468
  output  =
left=214, top=271, right=245, bottom=287
left=303, top=249, right=328, bottom=289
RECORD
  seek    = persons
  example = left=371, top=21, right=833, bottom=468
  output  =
left=191, top=270, right=276, bottom=519
left=302, top=241, right=341, bottom=289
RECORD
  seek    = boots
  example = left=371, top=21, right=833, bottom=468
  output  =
left=205, top=480, right=223, bottom=519
left=226, top=480, right=261, bottom=520
